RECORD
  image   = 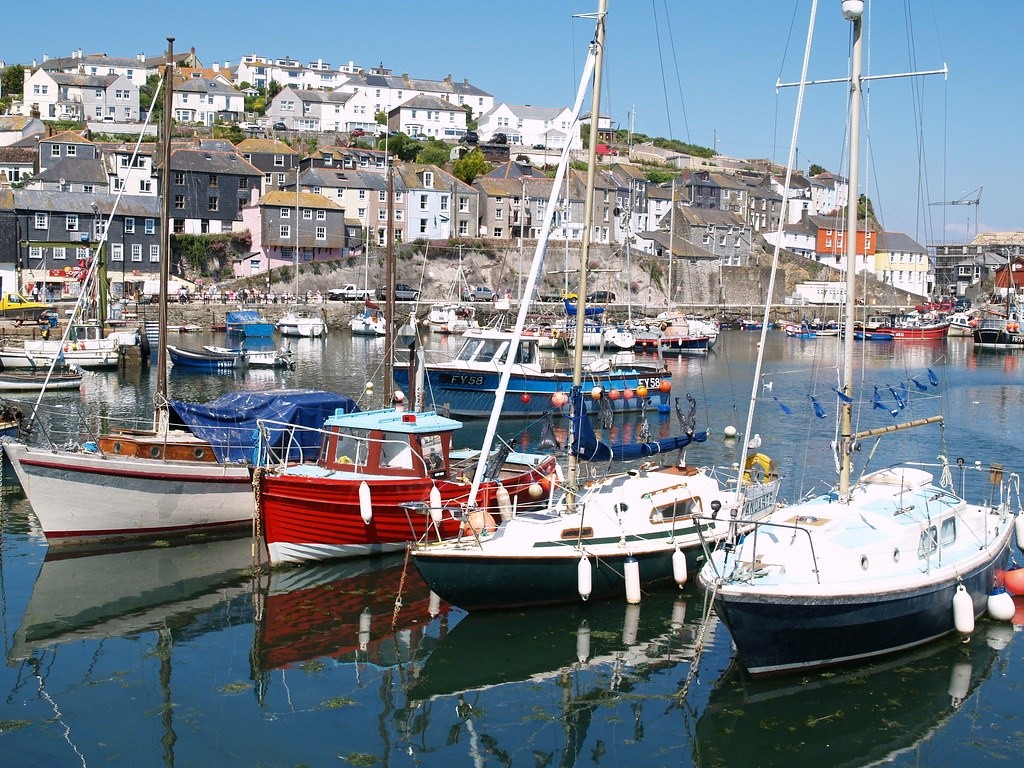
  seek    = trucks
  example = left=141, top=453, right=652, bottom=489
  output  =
left=328, top=284, right=376, bottom=301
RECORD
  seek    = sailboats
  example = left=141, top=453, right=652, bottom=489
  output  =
left=2, top=36, right=363, bottom=547
left=974, top=248, right=1024, bottom=349
left=462, top=176, right=723, bottom=357
left=398, top=0, right=785, bottom=612
left=247, top=149, right=564, bottom=569
left=693, top=0, right=1024, bottom=683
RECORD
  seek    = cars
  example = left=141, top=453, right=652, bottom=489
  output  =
left=586, top=290, right=616, bottom=303
left=375, top=283, right=422, bottom=301
left=464, top=285, right=500, bottom=301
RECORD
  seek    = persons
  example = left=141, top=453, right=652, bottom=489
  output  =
left=32, top=283, right=55, bottom=303
left=425, top=452, right=443, bottom=470
left=221, top=292, right=228, bottom=304
left=135, top=326, right=142, bottom=346
left=48, top=124, right=57, bottom=137
left=38, top=311, right=49, bottom=337
left=176, top=286, right=190, bottom=304
left=236, top=287, right=269, bottom=304
left=138, top=286, right=144, bottom=302
left=202, top=292, right=210, bottom=305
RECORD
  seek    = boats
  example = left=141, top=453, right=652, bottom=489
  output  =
left=276, top=304, right=328, bottom=337
left=166, top=344, right=298, bottom=371
left=841, top=318, right=950, bottom=341
